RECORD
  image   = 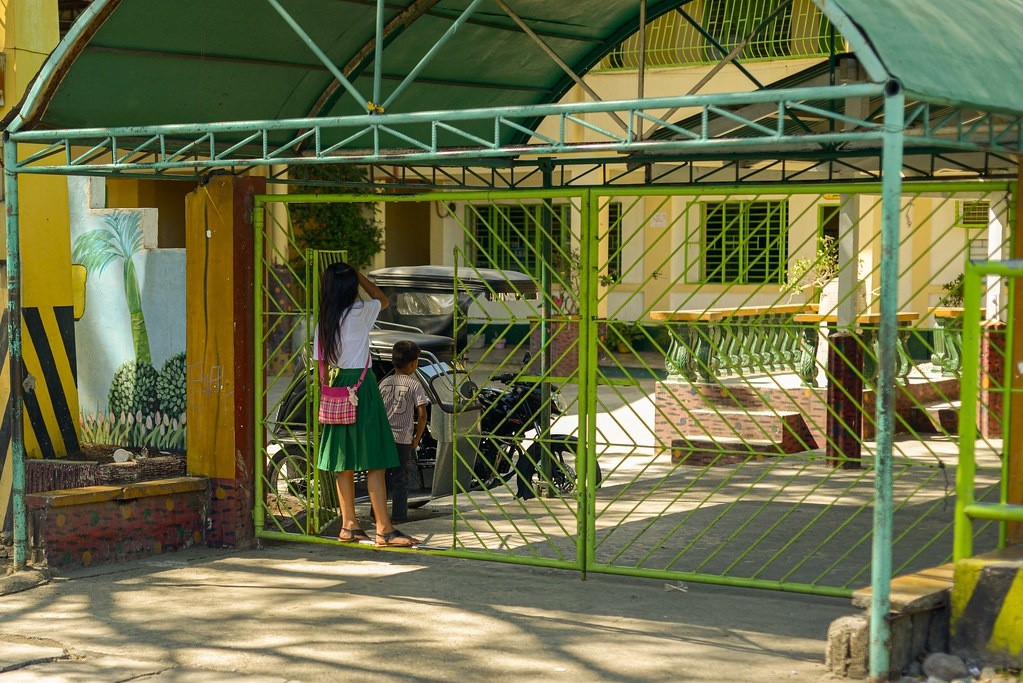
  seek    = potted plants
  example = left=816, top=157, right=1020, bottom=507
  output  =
left=602, top=319, right=644, bottom=355
left=789, top=238, right=838, bottom=293
left=465, top=326, right=487, bottom=348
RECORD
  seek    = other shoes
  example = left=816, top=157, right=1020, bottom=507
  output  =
left=370, top=517, right=376, bottom=522
left=391, top=519, right=408, bottom=524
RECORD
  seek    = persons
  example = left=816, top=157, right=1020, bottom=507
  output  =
left=370, top=339, right=429, bottom=525
left=312, top=261, right=422, bottom=547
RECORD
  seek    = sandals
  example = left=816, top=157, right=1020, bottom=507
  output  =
left=337, top=528, right=371, bottom=542
left=374, top=530, right=421, bottom=547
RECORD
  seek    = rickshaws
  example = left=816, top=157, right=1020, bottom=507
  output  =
left=263, top=270, right=604, bottom=510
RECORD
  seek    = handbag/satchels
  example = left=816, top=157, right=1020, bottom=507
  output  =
left=318, top=385, right=358, bottom=425
